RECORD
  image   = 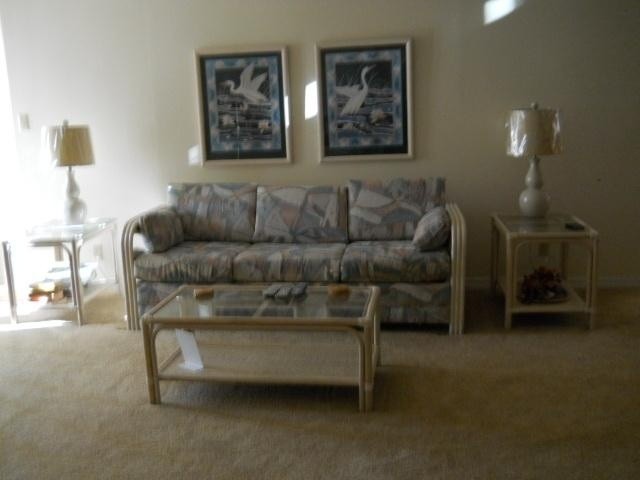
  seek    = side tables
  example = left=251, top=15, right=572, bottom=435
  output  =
left=2, top=217, right=119, bottom=326
left=489, top=212, right=598, bottom=330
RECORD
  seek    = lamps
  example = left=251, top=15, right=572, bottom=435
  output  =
left=48, top=119, right=94, bottom=227
left=506, top=101, right=563, bottom=217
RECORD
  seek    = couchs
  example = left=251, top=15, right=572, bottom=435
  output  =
left=120, top=204, right=467, bottom=334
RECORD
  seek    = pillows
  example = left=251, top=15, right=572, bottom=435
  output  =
left=166, top=183, right=258, bottom=244
left=253, top=184, right=349, bottom=244
left=140, top=205, right=183, bottom=252
left=348, top=177, right=445, bottom=240
left=412, top=206, right=451, bottom=251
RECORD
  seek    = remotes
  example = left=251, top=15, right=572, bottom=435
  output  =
left=292, top=282, right=307, bottom=295
left=263, top=284, right=281, bottom=296
left=276, top=285, right=291, bottom=296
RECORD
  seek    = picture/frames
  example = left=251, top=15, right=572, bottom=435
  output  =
left=192, top=45, right=292, bottom=168
left=316, top=35, right=414, bottom=163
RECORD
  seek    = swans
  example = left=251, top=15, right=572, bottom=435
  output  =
left=218, top=60, right=269, bottom=105
left=336, top=64, right=377, bottom=128
